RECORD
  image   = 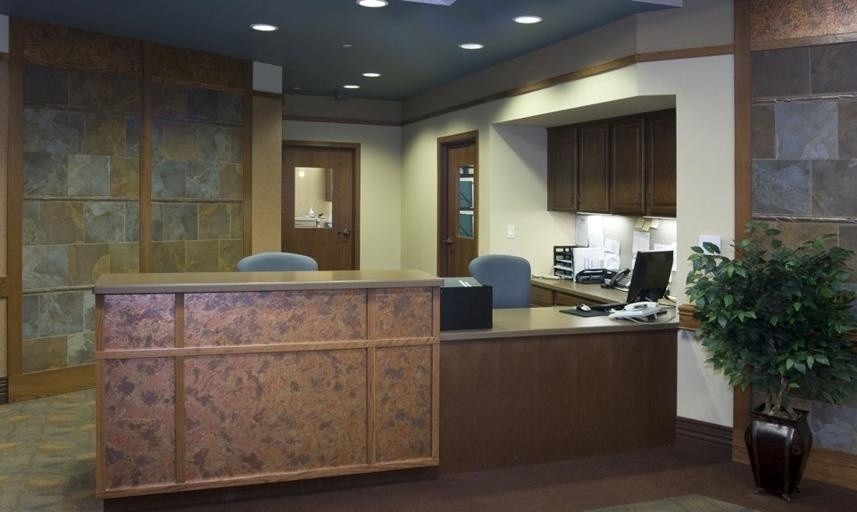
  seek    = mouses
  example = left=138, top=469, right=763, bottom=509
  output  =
left=577, top=303, right=592, bottom=313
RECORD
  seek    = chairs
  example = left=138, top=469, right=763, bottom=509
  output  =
left=235, top=252, right=318, bottom=271
left=467, top=254, right=531, bottom=308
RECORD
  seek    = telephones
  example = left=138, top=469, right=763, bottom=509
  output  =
left=610, top=268, right=633, bottom=292
left=609, top=301, right=668, bottom=325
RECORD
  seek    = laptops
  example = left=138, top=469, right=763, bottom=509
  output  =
left=593, top=250, right=675, bottom=312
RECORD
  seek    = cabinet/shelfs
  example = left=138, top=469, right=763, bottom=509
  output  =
left=547, top=120, right=611, bottom=212
left=612, top=108, right=676, bottom=217
left=531, top=285, right=554, bottom=307
left=556, top=292, right=603, bottom=306
left=553, top=244, right=589, bottom=280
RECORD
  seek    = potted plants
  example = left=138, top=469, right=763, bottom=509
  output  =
left=683, top=219, right=856, bottom=493
